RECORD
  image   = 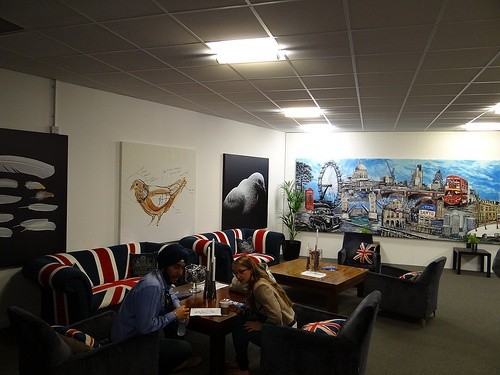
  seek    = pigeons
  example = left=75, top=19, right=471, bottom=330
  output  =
left=222, top=171, right=267, bottom=222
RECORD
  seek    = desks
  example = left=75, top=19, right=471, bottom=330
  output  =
left=269, top=258, right=370, bottom=314
left=453, top=247, right=491, bottom=278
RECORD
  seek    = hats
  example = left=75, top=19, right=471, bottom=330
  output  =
left=157, top=244, right=194, bottom=271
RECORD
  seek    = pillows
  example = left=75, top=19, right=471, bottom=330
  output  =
left=50, top=325, right=102, bottom=351
left=301, top=318, right=347, bottom=336
left=398, top=271, right=423, bottom=281
left=130, top=252, right=158, bottom=277
left=352, top=242, right=380, bottom=265
left=236, top=236, right=256, bottom=254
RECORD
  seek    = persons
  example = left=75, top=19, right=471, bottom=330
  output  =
left=110, top=243, right=203, bottom=375
left=224, top=256, right=297, bottom=375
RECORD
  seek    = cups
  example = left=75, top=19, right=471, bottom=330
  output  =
left=219, top=301, right=230, bottom=316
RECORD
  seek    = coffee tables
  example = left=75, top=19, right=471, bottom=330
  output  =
left=176, top=286, right=251, bottom=375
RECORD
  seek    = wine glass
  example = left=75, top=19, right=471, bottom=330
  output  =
left=185, top=265, right=205, bottom=293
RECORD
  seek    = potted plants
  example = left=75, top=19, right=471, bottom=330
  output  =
left=467, top=234, right=471, bottom=250
left=471, top=234, right=480, bottom=250
left=282, top=188, right=310, bottom=261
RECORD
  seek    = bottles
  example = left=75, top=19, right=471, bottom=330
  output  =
left=177, top=317, right=186, bottom=336
left=203, top=237, right=216, bottom=300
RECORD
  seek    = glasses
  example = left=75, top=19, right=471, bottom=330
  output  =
left=232, top=268, right=249, bottom=277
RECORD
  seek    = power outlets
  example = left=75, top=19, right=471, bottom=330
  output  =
left=51, top=125, right=59, bottom=134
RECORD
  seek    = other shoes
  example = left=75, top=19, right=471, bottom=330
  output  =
left=183, top=357, right=202, bottom=368
left=225, top=360, right=239, bottom=369
left=225, top=368, right=250, bottom=375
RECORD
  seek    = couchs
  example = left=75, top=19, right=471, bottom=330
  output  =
left=338, top=232, right=380, bottom=287
left=22, top=242, right=197, bottom=329
left=364, top=257, right=447, bottom=329
left=179, top=228, right=285, bottom=285
left=6, top=304, right=160, bottom=375
left=258, top=289, right=382, bottom=375
